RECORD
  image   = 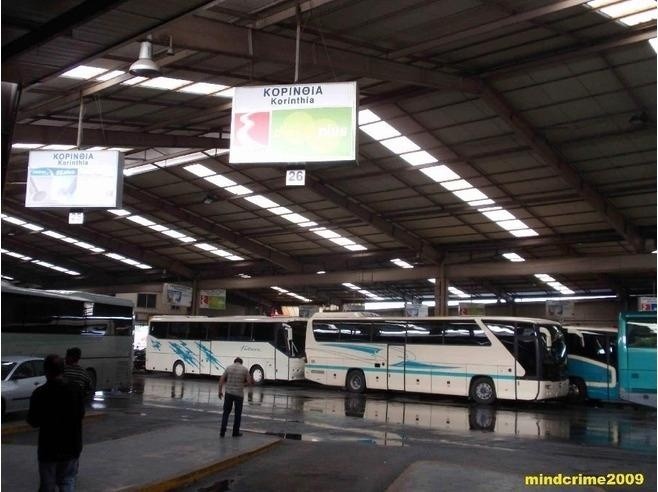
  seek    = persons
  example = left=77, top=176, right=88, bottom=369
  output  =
left=63, top=347, right=96, bottom=474
left=218, top=357, right=253, bottom=437
left=26, top=354, right=90, bottom=491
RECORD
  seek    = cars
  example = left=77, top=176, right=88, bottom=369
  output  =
left=1, top=352, right=48, bottom=418
left=134, top=347, right=146, bottom=369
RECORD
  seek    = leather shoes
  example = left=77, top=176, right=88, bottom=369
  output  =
left=220, top=434, right=225, bottom=437
left=232, top=431, right=243, bottom=437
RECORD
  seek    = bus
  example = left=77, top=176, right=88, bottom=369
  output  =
left=298, top=391, right=578, bottom=446
left=0, top=280, right=136, bottom=395
left=143, top=304, right=656, bottom=412
left=141, top=372, right=298, bottom=411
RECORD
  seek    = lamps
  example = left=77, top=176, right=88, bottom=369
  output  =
left=127, top=37, right=163, bottom=80
